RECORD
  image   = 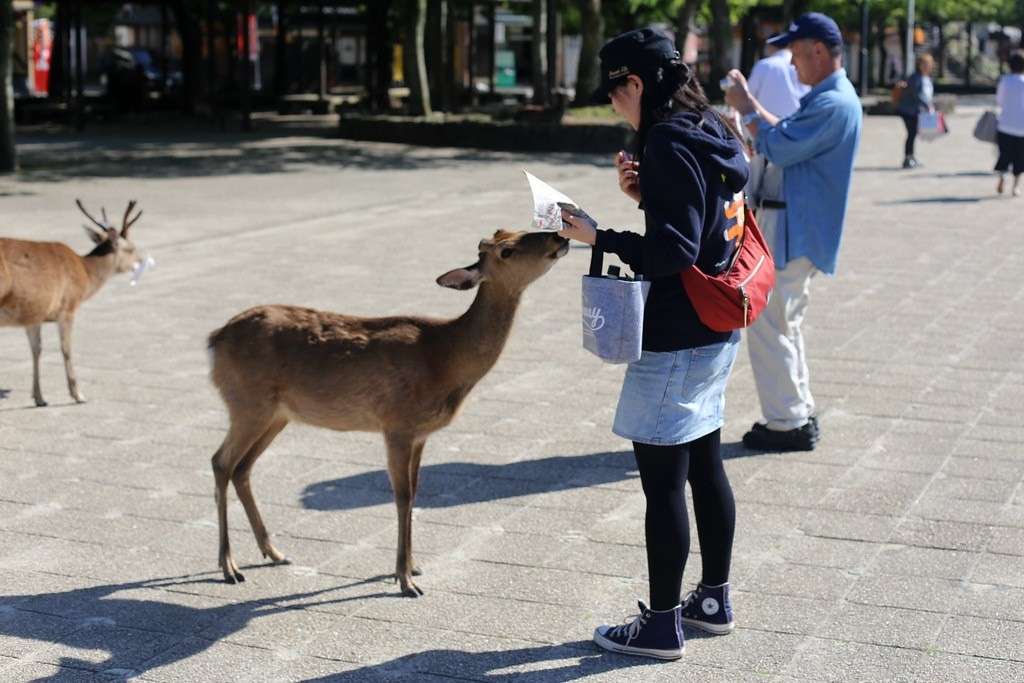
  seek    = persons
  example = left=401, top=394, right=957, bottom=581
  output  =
left=555, top=27, right=750, bottom=660
left=898, top=53, right=936, bottom=169
left=725, top=12, right=863, bottom=452
left=994, top=54, right=1024, bottom=197
left=743, top=33, right=812, bottom=124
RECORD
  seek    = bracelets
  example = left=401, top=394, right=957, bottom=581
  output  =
left=741, top=109, right=761, bottom=127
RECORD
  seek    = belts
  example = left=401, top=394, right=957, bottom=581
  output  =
left=755, top=195, right=788, bottom=210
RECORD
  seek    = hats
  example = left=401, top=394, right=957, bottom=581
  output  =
left=766, top=11, right=843, bottom=47
left=585, top=27, right=680, bottom=104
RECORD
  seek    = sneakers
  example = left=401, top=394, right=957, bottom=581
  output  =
left=593, top=599, right=687, bottom=659
left=680, top=581, right=734, bottom=634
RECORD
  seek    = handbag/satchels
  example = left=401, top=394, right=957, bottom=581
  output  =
left=680, top=210, right=776, bottom=332
left=916, top=111, right=949, bottom=141
left=891, top=80, right=908, bottom=109
left=582, top=229, right=650, bottom=364
left=974, top=111, right=998, bottom=144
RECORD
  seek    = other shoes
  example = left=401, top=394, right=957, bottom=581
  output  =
left=1013, top=189, right=1022, bottom=196
left=752, top=416, right=820, bottom=441
left=997, top=178, right=1005, bottom=193
left=742, top=423, right=816, bottom=451
left=902, top=158, right=922, bottom=168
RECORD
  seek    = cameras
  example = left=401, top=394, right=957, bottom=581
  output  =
left=719, top=76, right=736, bottom=90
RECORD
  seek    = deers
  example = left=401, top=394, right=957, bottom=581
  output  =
left=0, top=198, right=146, bottom=403
left=206, top=229, right=570, bottom=598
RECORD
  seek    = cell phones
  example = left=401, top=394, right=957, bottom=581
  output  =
left=620, top=150, right=640, bottom=185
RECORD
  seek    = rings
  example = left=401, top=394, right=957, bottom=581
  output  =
left=564, top=237, right=567, bottom=239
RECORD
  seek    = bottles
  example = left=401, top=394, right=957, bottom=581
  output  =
left=127, top=256, right=154, bottom=286
left=607, top=265, right=620, bottom=279
